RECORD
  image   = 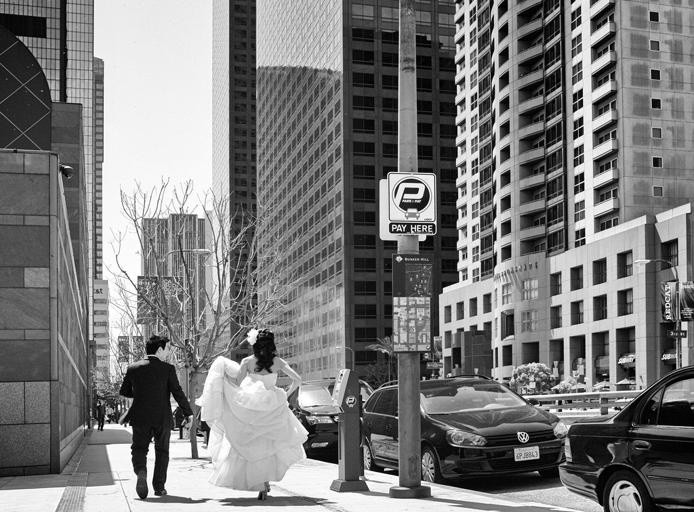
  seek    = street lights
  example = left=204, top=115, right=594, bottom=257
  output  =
left=634, top=259, right=680, bottom=368
left=154, top=246, right=211, bottom=330
left=336, top=345, right=355, bottom=372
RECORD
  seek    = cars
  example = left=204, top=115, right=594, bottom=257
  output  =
left=362, top=374, right=568, bottom=486
left=560, top=363, right=694, bottom=512
left=288, top=377, right=375, bottom=459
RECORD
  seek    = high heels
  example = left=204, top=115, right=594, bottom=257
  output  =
left=258, top=482, right=271, bottom=500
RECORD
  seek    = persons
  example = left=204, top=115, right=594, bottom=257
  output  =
left=95, top=399, right=106, bottom=431
left=213, top=328, right=302, bottom=502
left=116, top=334, right=194, bottom=499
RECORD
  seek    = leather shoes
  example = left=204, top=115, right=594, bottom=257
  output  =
left=136, top=470, right=148, bottom=498
left=154, top=489, right=167, bottom=496
